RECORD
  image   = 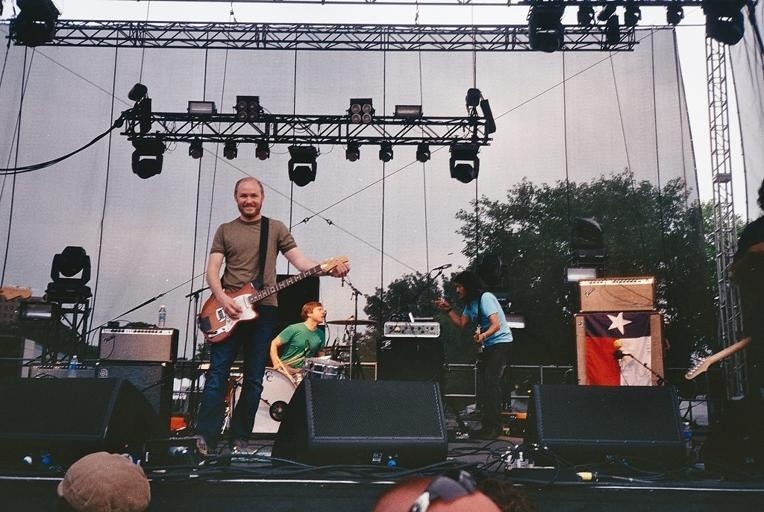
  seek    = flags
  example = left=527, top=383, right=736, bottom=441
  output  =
left=585, top=311, right=652, bottom=386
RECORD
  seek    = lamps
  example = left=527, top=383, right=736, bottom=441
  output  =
left=578, top=3, right=642, bottom=45
left=7, top=0, right=61, bottom=48
left=667, top=5, right=683, bottom=24
left=572, top=218, right=604, bottom=261
left=526, top=4, right=564, bottom=52
left=47, top=247, right=90, bottom=287
left=703, top=9, right=746, bottom=46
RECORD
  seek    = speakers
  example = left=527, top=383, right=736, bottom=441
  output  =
left=523, top=385, right=687, bottom=470
left=0, top=377, right=159, bottom=466
left=273, top=378, right=449, bottom=470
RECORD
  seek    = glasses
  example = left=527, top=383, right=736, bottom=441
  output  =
left=409, top=468, right=479, bottom=512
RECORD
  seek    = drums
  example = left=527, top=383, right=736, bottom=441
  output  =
left=194, top=364, right=240, bottom=407
left=230, top=366, right=297, bottom=434
left=307, top=359, right=343, bottom=381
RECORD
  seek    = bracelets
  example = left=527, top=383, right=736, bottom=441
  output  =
left=447, top=307, right=452, bottom=313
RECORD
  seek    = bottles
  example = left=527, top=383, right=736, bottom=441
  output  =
left=158, top=305, right=166, bottom=328
left=682, top=421, right=693, bottom=449
left=69, top=355, right=78, bottom=369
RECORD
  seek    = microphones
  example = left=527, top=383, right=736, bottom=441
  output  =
left=433, top=263, right=452, bottom=270
left=323, top=310, right=328, bottom=327
left=341, top=276, right=345, bottom=288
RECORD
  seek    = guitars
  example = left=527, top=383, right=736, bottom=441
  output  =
left=198, top=257, right=349, bottom=343
left=472, top=325, right=484, bottom=354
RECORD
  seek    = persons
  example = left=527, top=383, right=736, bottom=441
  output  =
left=57, top=452, right=151, bottom=512
left=269, top=302, right=325, bottom=375
left=375, top=477, right=501, bottom=512
left=435, top=272, right=514, bottom=440
left=733, top=180, right=764, bottom=399
left=195, top=178, right=350, bottom=456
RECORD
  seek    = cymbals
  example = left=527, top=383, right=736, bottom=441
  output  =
left=323, top=319, right=379, bottom=325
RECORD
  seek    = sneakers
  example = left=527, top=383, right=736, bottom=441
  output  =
left=231, top=441, right=242, bottom=456
left=194, top=437, right=217, bottom=456
